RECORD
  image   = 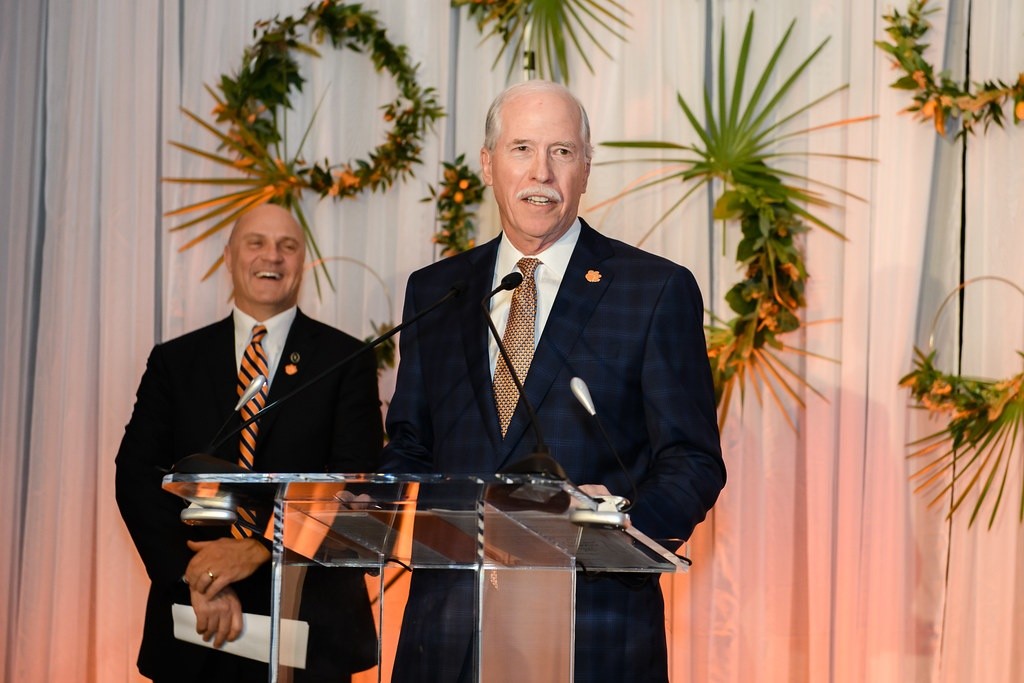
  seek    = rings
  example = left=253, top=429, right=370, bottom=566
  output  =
left=207, top=570, right=215, bottom=581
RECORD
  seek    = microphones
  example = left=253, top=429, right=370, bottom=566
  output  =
left=168, top=279, right=471, bottom=495
left=481, top=272, right=572, bottom=513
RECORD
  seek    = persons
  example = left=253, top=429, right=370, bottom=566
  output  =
left=114, top=204, right=387, bottom=683
left=323, top=78, right=729, bottom=683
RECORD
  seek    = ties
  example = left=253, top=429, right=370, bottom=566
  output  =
left=230, top=325, right=268, bottom=539
left=492, top=258, right=544, bottom=440
